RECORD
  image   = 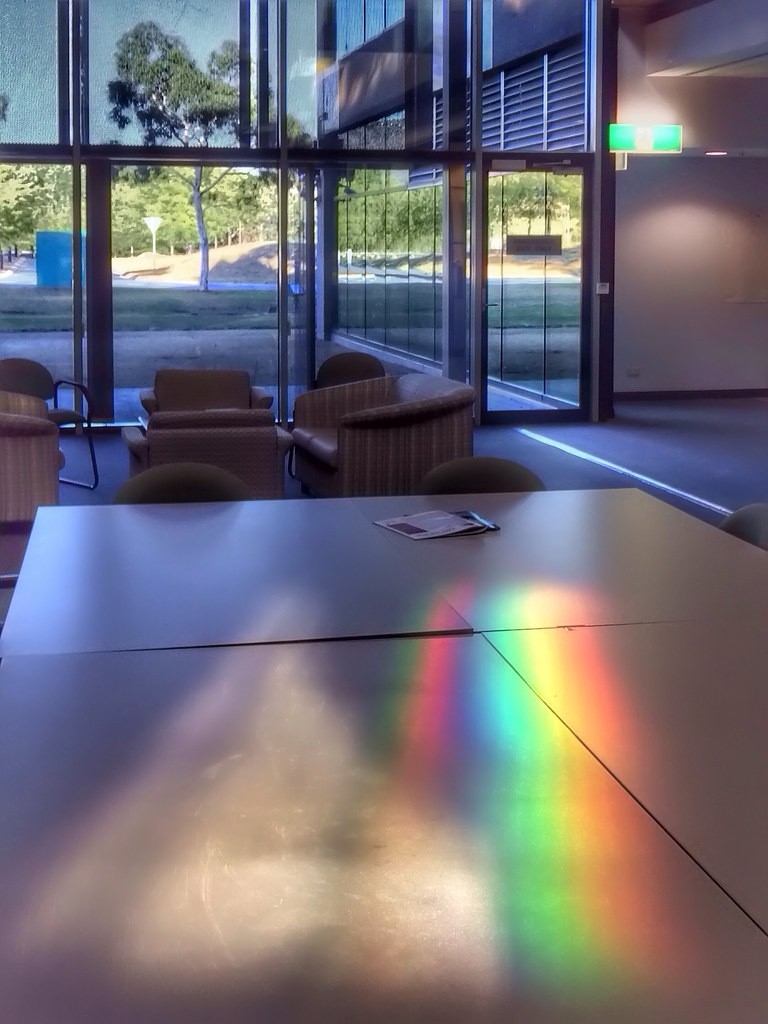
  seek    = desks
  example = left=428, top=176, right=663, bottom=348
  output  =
left=1, top=487, right=768, bottom=1024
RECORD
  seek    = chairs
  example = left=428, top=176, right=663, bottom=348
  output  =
left=288, top=351, right=384, bottom=479
left=417, top=458, right=546, bottom=496
left=0, top=357, right=56, bottom=400
left=718, top=503, right=767, bottom=553
left=112, top=462, right=256, bottom=506
left=47, top=379, right=100, bottom=490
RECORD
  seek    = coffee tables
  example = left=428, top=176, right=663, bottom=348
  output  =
left=138, top=416, right=149, bottom=431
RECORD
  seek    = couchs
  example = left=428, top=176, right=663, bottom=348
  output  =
left=139, top=369, right=274, bottom=416
left=291, top=374, right=478, bottom=497
left=0, top=391, right=65, bottom=523
left=120, top=408, right=293, bottom=503
